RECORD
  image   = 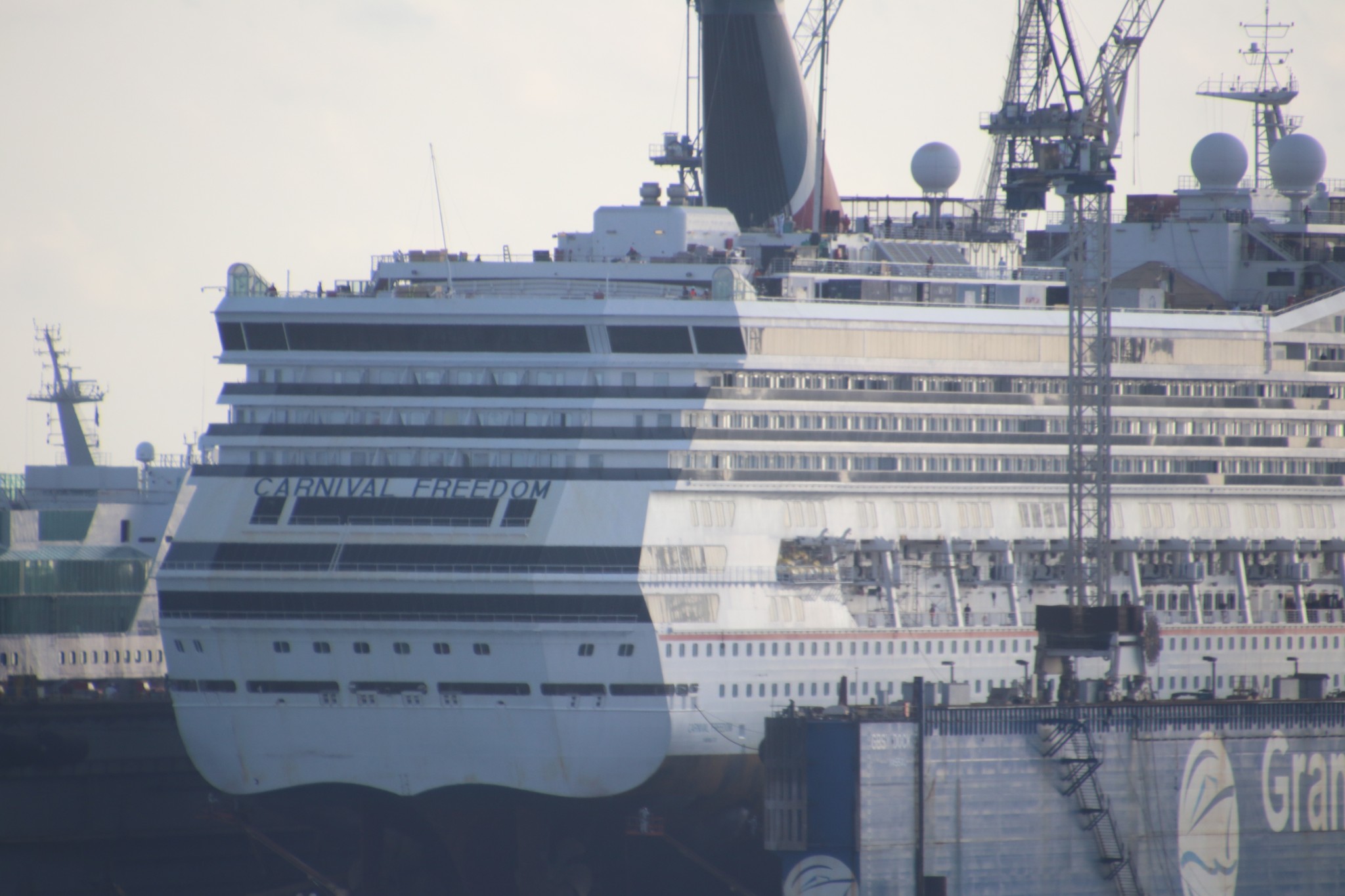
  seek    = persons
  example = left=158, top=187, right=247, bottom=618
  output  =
left=996, top=257, right=1006, bottom=278
left=267, top=283, right=278, bottom=297
left=682, top=285, right=710, bottom=299
left=1137, top=200, right=1341, bottom=312
left=840, top=207, right=979, bottom=236
left=317, top=281, right=325, bottom=297
left=473, top=253, right=482, bottom=263
left=626, top=247, right=639, bottom=263
left=926, top=256, right=934, bottom=277
left=392, top=249, right=405, bottom=263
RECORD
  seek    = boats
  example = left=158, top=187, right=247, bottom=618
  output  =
left=0, top=326, right=191, bottom=725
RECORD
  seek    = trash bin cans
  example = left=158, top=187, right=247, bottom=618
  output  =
left=1293, top=674, right=1329, bottom=700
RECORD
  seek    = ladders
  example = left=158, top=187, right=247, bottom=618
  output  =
left=503, top=245, right=511, bottom=262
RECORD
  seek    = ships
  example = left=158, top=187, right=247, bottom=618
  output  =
left=153, top=1, right=1345, bottom=896
